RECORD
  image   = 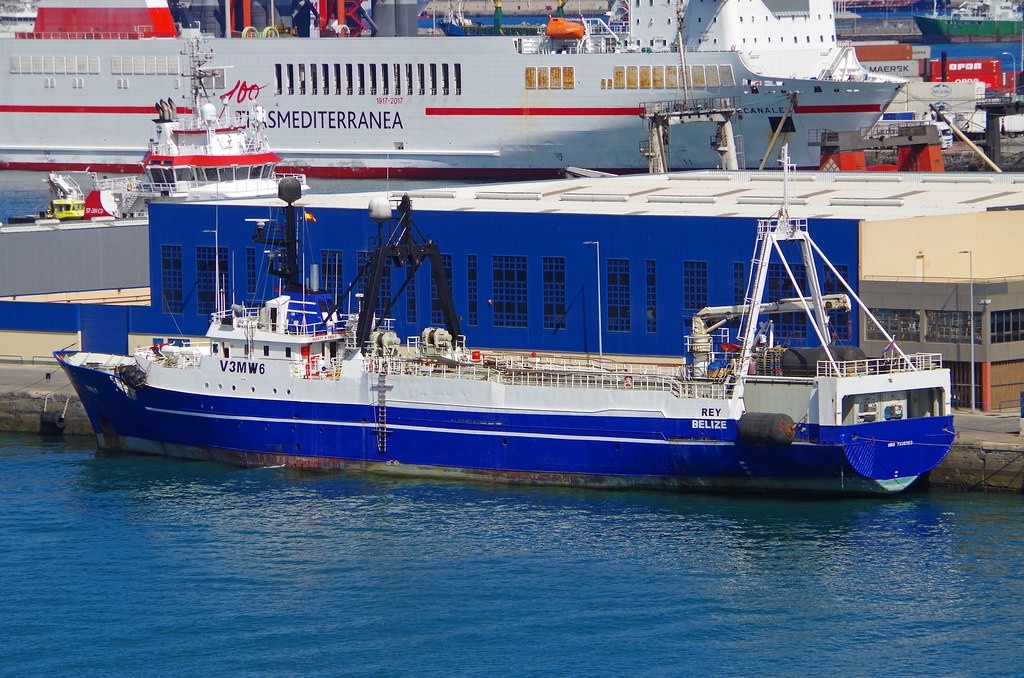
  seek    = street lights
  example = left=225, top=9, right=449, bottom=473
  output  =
left=582, top=241, right=602, bottom=358
left=959, top=251, right=976, bottom=413
left=1002, top=52, right=1016, bottom=94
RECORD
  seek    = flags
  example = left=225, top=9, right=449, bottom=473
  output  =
left=304, top=210, right=316, bottom=222
left=880, top=341, right=895, bottom=353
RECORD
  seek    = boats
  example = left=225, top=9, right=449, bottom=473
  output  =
left=42, top=170, right=85, bottom=219
left=0, top=0, right=38, bottom=39
left=0, top=0, right=952, bottom=171
left=54, top=19, right=956, bottom=494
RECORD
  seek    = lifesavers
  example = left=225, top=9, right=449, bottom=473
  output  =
left=624, top=376, right=633, bottom=388
